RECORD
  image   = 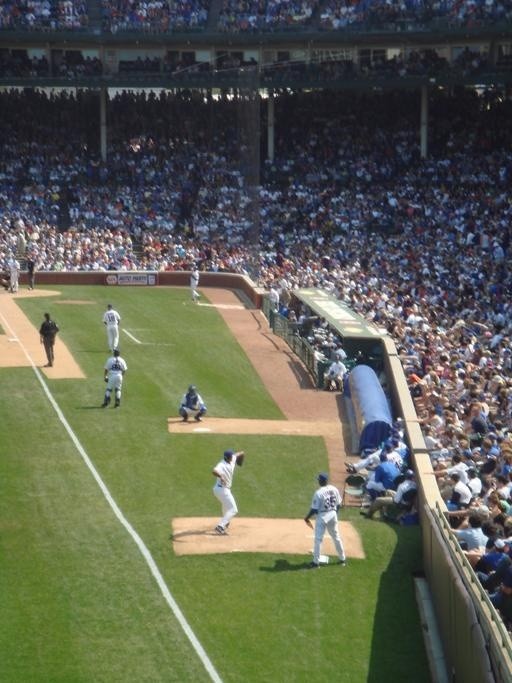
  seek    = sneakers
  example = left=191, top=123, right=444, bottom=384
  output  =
left=309, top=563, right=320, bottom=568
left=215, top=526, right=226, bottom=535
left=345, top=462, right=355, bottom=474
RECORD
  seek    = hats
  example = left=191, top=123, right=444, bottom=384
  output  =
left=318, top=474, right=327, bottom=480
left=224, top=451, right=232, bottom=456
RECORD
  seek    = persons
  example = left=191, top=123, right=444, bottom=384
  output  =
left=38, top=312, right=60, bottom=367
left=102, top=303, right=121, bottom=353
left=101, top=349, right=128, bottom=407
left=211, top=448, right=246, bottom=535
left=249, top=293, right=512, bottom=649
left=1, top=2, right=511, bottom=293
left=188, top=264, right=201, bottom=302
left=177, top=381, right=207, bottom=422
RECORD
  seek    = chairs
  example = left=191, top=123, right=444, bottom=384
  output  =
left=343, top=473, right=365, bottom=510
left=385, top=487, right=418, bottom=524
